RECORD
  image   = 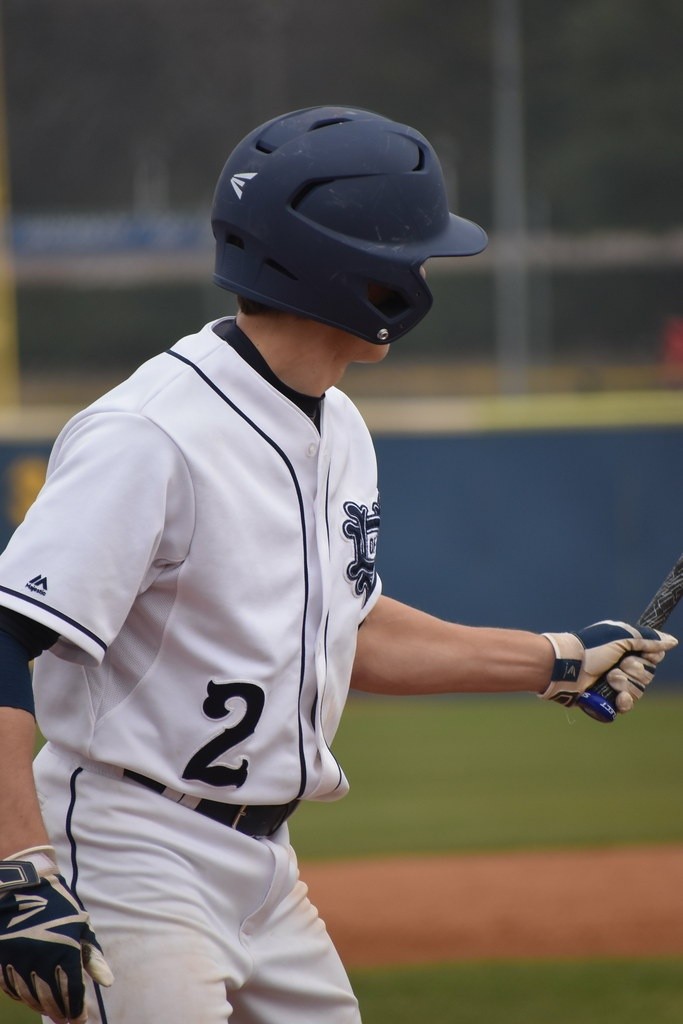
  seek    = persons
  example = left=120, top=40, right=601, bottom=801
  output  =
left=0, top=106, right=678, bottom=1024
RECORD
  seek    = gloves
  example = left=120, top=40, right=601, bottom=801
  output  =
left=536, top=619, right=678, bottom=715
left=0, top=845, right=118, bottom=1023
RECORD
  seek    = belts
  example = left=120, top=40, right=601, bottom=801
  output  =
left=115, top=761, right=309, bottom=837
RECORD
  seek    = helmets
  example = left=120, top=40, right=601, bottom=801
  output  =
left=206, top=103, right=490, bottom=348
